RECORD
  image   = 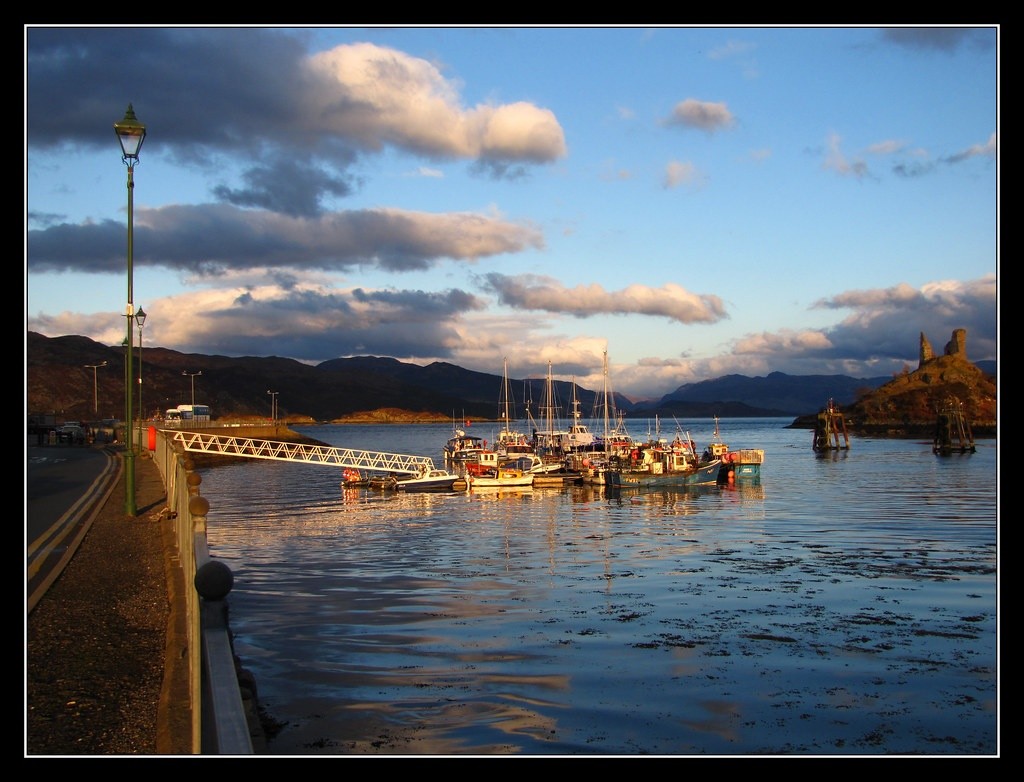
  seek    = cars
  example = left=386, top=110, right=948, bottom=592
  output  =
left=55, top=426, right=84, bottom=444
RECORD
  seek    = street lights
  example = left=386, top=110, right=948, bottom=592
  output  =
left=112, top=100, right=147, bottom=517
left=267, top=390, right=280, bottom=419
left=84, top=361, right=108, bottom=413
left=183, top=371, right=203, bottom=404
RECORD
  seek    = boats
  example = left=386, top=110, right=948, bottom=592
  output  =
left=338, top=349, right=766, bottom=494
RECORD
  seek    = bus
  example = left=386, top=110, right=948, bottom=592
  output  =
left=177, top=404, right=210, bottom=422
